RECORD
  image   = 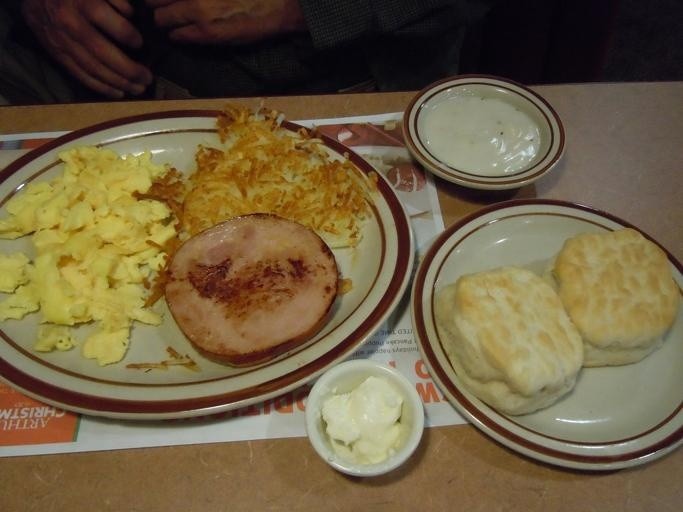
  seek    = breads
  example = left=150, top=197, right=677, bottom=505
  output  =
left=436, top=267, right=583, bottom=417
left=545, top=226, right=680, bottom=365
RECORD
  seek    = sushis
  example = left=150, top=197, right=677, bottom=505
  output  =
left=163, top=212, right=342, bottom=363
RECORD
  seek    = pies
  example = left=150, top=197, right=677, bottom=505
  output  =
left=145, top=100, right=370, bottom=305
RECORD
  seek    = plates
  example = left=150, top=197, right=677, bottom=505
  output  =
left=411, top=198, right=683, bottom=474
left=400, top=75, right=567, bottom=192
left=0, top=110, right=415, bottom=420
left=304, top=359, right=425, bottom=479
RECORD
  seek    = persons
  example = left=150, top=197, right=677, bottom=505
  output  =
left=5, top=0, right=493, bottom=100
